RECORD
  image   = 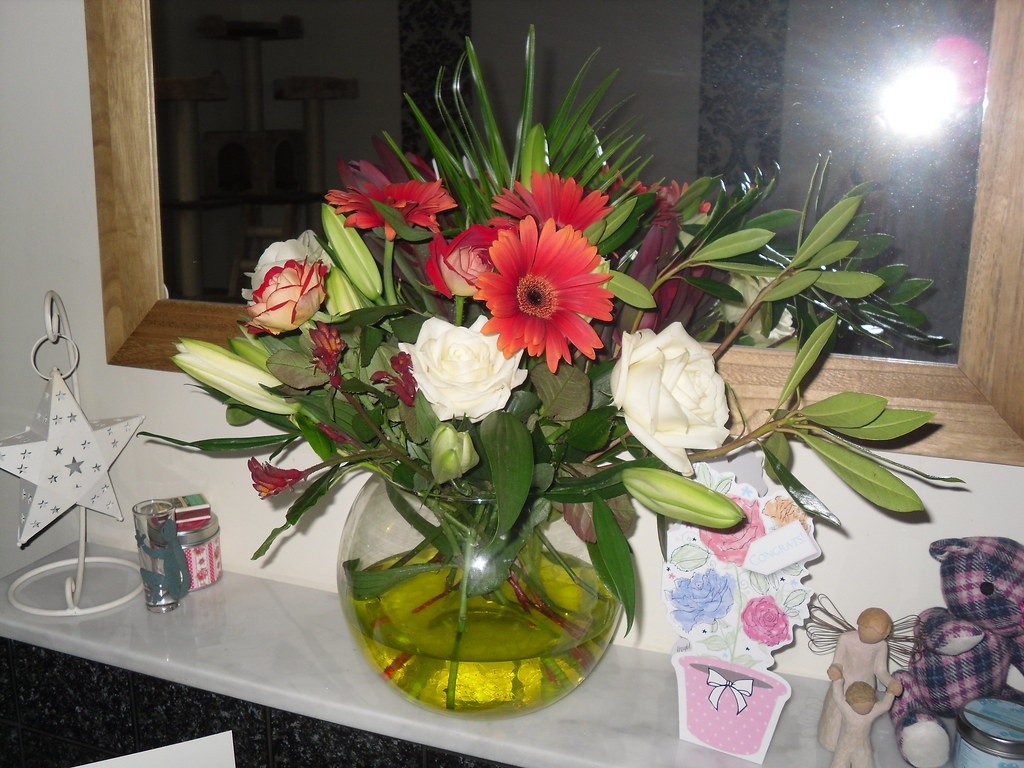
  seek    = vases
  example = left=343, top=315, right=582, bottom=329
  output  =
left=333, top=465, right=623, bottom=716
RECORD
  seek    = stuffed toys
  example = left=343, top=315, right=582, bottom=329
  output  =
left=884, top=536, right=1024, bottom=768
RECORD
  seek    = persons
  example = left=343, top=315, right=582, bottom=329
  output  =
left=821, top=608, right=902, bottom=768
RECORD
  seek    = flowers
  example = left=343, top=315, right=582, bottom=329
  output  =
left=133, top=23, right=967, bottom=705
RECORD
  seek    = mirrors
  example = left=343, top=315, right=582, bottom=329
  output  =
left=85, top=0, right=1024, bottom=467
